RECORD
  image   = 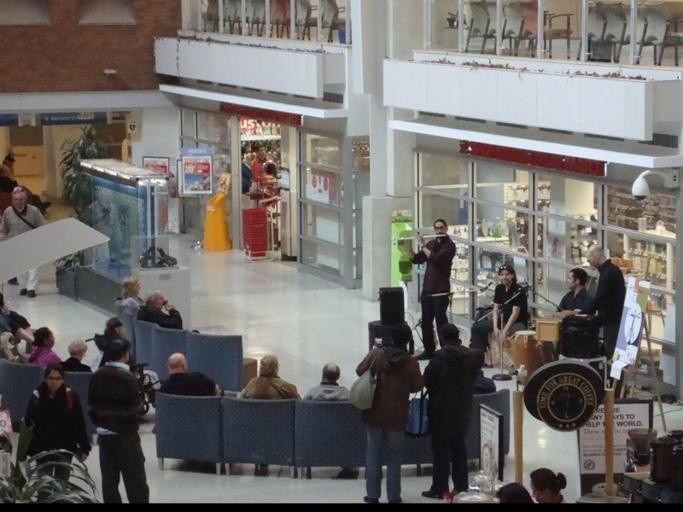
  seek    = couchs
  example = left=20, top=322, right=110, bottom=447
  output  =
left=295, top=398, right=419, bottom=478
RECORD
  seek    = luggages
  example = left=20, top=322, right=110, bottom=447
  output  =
left=561, top=313, right=599, bottom=360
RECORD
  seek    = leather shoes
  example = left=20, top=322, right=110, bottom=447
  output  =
left=387, top=497, right=402, bottom=503
left=363, top=496, right=379, bottom=505
left=26, top=290, right=36, bottom=298
left=19, top=289, right=27, bottom=295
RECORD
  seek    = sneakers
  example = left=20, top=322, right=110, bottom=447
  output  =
left=416, top=351, right=432, bottom=360
left=40, top=201, right=51, bottom=214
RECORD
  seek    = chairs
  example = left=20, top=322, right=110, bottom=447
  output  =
left=204, top=1, right=351, bottom=44
left=63, top=372, right=97, bottom=436
left=463, top=388, right=509, bottom=461
left=465, top=0, right=682, bottom=66
left=154, top=391, right=222, bottom=471
left=185, top=330, right=257, bottom=390
left=123, top=311, right=136, bottom=350
left=222, top=397, right=296, bottom=477
left=1, top=359, right=41, bottom=424
left=413, top=397, right=434, bottom=476
left=133, top=317, right=158, bottom=370
left=151, top=326, right=193, bottom=381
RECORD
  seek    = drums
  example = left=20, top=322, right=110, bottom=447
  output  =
left=514, top=330, right=541, bottom=385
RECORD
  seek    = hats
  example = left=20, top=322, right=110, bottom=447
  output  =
left=5, top=156, right=16, bottom=161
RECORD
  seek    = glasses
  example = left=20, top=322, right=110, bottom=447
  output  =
left=434, top=225, right=446, bottom=229
left=500, top=265, right=511, bottom=271
left=46, top=375, right=63, bottom=382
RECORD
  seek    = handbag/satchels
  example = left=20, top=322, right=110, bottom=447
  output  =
left=68, top=424, right=93, bottom=462
left=0, top=409, right=13, bottom=436
left=348, top=371, right=378, bottom=411
left=406, top=398, right=429, bottom=436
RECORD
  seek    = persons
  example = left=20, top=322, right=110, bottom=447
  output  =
left=355, top=320, right=426, bottom=504
left=469, top=348, right=496, bottom=394
left=160, top=353, right=219, bottom=396
left=114, top=276, right=183, bottom=330
left=305, top=363, right=350, bottom=400
left=555, top=268, right=596, bottom=364
left=239, top=141, right=277, bottom=207
left=578, top=244, right=627, bottom=386
left=1, top=154, right=51, bottom=216
left=1, top=187, right=46, bottom=297
left=241, top=355, right=302, bottom=401
left=421, top=322, right=473, bottom=499
left=1, top=295, right=150, bottom=504
left=529, top=467, right=567, bottom=503
left=470, top=264, right=527, bottom=374
left=496, top=482, right=532, bottom=505
left=403, top=219, right=456, bottom=360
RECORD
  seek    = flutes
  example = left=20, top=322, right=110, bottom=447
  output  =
left=398, top=234, right=446, bottom=241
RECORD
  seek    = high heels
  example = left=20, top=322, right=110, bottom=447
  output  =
left=421, top=488, right=448, bottom=499
